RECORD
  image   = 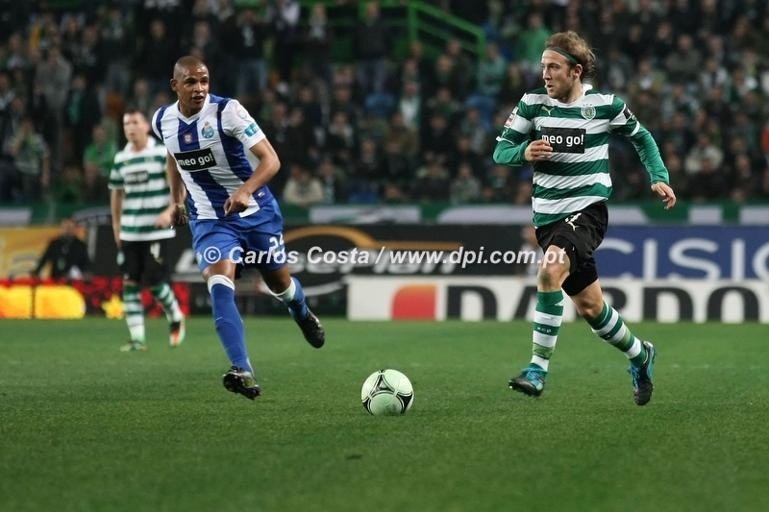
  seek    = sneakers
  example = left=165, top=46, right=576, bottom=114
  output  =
left=119, top=340, right=147, bottom=352
left=627, top=341, right=657, bottom=405
left=288, top=305, right=325, bottom=348
left=508, top=370, right=545, bottom=396
left=223, top=365, right=260, bottom=400
left=169, top=314, right=186, bottom=347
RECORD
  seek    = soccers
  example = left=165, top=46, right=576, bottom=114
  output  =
left=361, top=368, right=414, bottom=416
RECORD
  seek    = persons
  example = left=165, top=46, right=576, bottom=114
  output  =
left=0, top=0, right=768, bottom=206
left=108, top=107, right=189, bottom=353
left=149, top=55, right=326, bottom=400
left=35, top=214, right=89, bottom=281
left=491, top=29, right=678, bottom=407
